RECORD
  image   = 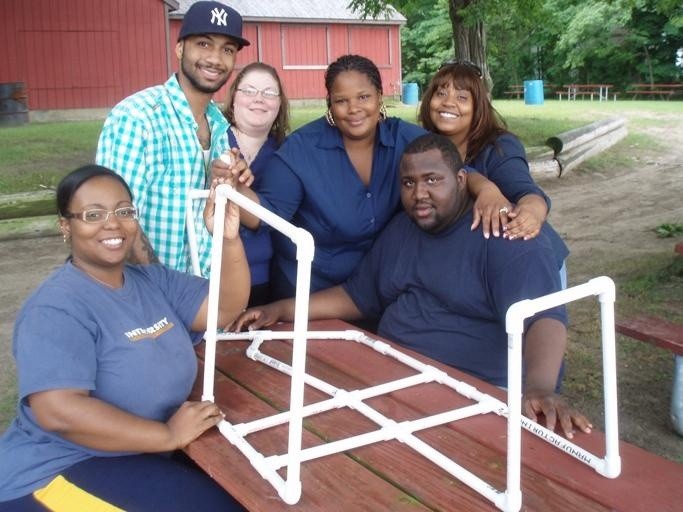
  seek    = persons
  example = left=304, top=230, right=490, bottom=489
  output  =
left=413, top=57, right=551, bottom=241
left=212, top=61, right=289, bottom=311
left=93, top=1, right=250, bottom=346
left=219, top=135, right=597, bottom=443
left=203, top=53, right=515, bottom=327
left=0, top=162, right=254, bottom=511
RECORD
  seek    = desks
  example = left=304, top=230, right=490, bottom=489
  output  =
left=181, top=318, right=683, bottom=512
left=509, top=83, right=683, bottom=102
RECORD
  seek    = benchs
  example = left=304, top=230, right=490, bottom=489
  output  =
left=611, top=313, right=683, bottom=436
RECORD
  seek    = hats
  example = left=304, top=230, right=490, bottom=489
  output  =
left=177, top=0, right=251, bottom=51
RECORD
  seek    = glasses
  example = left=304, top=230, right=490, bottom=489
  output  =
left=236, top=87, right=282, bottom=98
left=66, top=207, right=137, bottom=224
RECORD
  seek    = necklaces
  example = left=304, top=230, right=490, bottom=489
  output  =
left=87, top=272, right=124, bottom=290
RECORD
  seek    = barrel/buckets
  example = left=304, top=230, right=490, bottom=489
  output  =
left=0, top=81, right=29, bottom=127
left=402, top=82, right=419, bottom=105
left=523, top=79, right=545, bottom=104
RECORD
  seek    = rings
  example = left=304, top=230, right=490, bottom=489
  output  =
left=500, top=207, right=508, bottom=213
